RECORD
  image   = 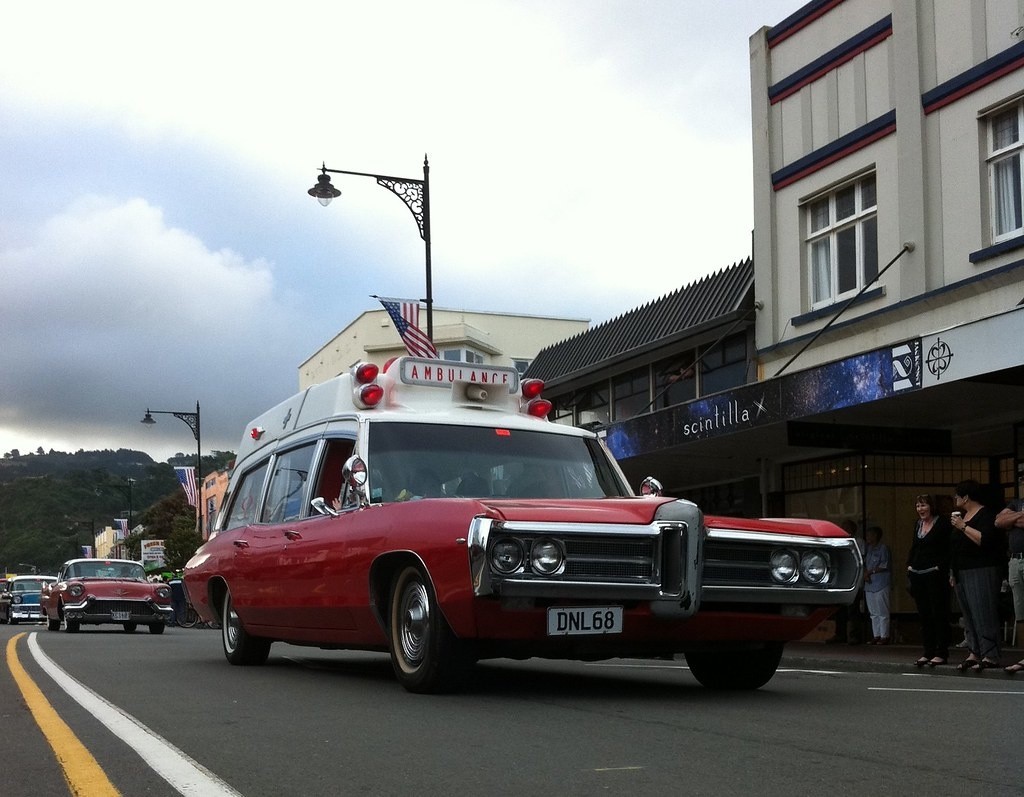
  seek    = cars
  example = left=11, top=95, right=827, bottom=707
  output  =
left=0, top=575, right=59, bottom=625
left=182, top=356, right=866, bottom=698
left=40, top=558, right=174, bottom=635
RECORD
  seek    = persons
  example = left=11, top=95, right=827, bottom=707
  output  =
left=951, top=478, right=1001, bottom=669
left=905, top=494, right=949, bottom=665
left=117, top=566, right=130, bottom=577
left=862, top=526, right=890, bottom=644
left=505, top=460, right=559, bottom=497
left=994, top=498, right=1024, bottom=672
left=841, top=520, right=864, bottom=555
left=332, top=498, right=342, bottom=510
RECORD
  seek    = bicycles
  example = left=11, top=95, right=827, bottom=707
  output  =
left=175, top=568, right=223, bottom=630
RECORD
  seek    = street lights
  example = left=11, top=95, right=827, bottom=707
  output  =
left=307, top=152, right=434, bottom=341
left=139, top=400, right=202, bottom=545
left=68, top=518, right=95, bottom=558
left=94, top=479, right=132, bottom=533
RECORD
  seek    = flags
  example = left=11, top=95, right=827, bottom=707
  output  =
left=117, top=521, right=128, bottom=537
left=83, top=546, right=92, bottom=558
left=174, top=466, right=198, bottom=508
left=379, top=297, right=438, bottom=358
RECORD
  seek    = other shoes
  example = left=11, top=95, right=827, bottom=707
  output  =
left=867, top=637, right=880, bottom=645
left=914, top=658, right=930, bottom=665
left=927, top=660, right=946, bottom=665
left=956, top=640, right=968, bottom=647
left=877, top=638, right=889, bottom=645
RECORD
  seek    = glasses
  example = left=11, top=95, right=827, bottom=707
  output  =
left=916, top=494, right=931, bottom=500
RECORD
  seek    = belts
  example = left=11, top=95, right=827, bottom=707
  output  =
left=1010, top=553, right=1024, bottom=559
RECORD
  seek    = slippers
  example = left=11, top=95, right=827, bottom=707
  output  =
left=1004, top=662, right=1024, bottom=671
left=957, top=659, right=978, bottom=669
left=972, top=661, right=1000, bottom=670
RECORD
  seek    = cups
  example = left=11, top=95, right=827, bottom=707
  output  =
left=952, top=511, right=961, bottom=517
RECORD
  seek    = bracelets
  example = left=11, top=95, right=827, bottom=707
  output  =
left=873, top=568, right=875, bottom=573
left=961, top=524, right=967, bottom=532
